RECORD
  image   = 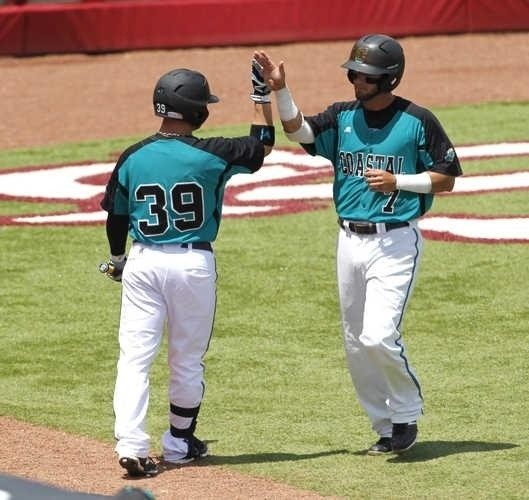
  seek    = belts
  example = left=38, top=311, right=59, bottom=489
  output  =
left=338, top=218, right=410, bottom=234
left=180, top=240, right=213, bottom=253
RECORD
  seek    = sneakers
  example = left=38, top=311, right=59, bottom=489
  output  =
left=169, top=443, right=209, bottom=464
left=391, top=420, right=419, bottom=454
left=367, top=436, right=394, bottom=456
left=119, top=456, right=159, bottom=478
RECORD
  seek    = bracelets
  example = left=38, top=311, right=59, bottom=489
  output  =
left=254, top=100, right=272, bottom=106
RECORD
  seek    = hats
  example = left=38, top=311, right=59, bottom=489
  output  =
left=340, top=33, right=406, bottom=77
left=152, top=68, right=219, bottom=125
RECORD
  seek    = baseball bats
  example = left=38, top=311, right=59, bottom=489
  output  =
left=99, top=262, right=116, bottom=274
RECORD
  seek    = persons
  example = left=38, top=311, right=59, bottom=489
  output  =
left=252, top=34, right=464, bottom=456
left=97, top=55, right=275, bottom=477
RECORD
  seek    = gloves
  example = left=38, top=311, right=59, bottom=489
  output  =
left=106, top=253, right=128, bottom=283
left=249, top=58, right=273, bottom=105
